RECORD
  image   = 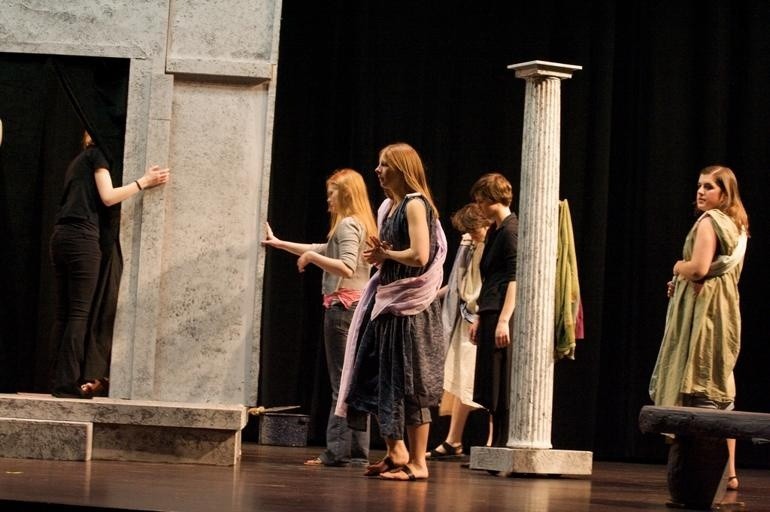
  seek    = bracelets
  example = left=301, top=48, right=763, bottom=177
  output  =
left=133, top=180, right=142, bottom=191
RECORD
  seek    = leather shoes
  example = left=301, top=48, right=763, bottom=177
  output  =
left=53, top=389, right=92, bottom=400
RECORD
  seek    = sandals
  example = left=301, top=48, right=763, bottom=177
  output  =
left=726, top=478, right=741, bottom=492
left=426, top=441, right=466, bottom=460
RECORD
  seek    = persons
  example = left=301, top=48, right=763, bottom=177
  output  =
left=654, top=166, right=750, bottom=493
left=261, top=168, right=377, bottom=470
left=468, top=172, right=521, bottom=471
left=427, top=201, right=498, bottom=463
left=48, top=118, right=170, bottom=401
left=342, top=144, right=450, bottom=482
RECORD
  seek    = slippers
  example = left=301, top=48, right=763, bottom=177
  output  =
left=367, top=456, right=404, bottom=476
left=380, top=464, right=429, bottom=481
left=304, top=457, right=326, bottom=467
